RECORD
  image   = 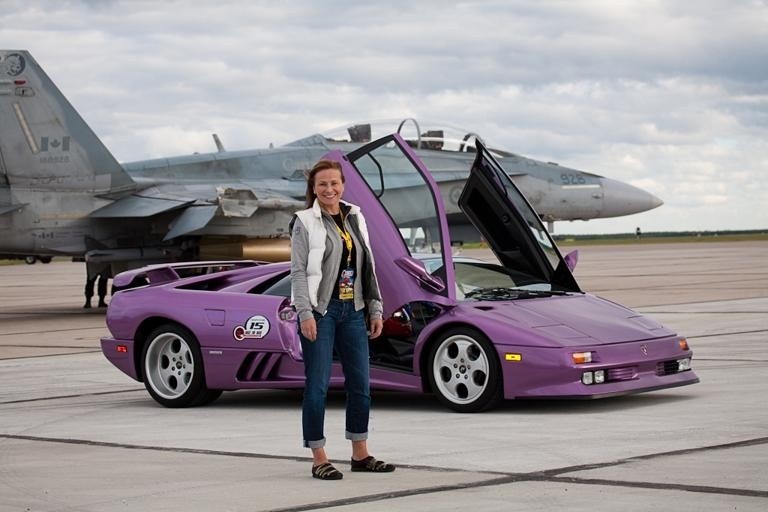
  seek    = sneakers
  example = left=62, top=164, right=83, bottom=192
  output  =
left=84, top=302, right=108, bottom=308
left=351, top=456, right=395, bottom=472
left=312, top=463, right=343, bottom=480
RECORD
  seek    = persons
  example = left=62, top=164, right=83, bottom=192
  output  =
left=479, top=235, right=485, bottom=248
left=82, top=267, right=109, bottom=308
left=288, top=158, right=398, bottom=481
left=635, top=226, right=642, bottom=239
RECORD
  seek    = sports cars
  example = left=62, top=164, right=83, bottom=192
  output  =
left=101, top=131, right=695, bottom=411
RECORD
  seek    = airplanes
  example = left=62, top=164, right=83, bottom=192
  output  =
left=0, top=47, right=663, bottom=284
